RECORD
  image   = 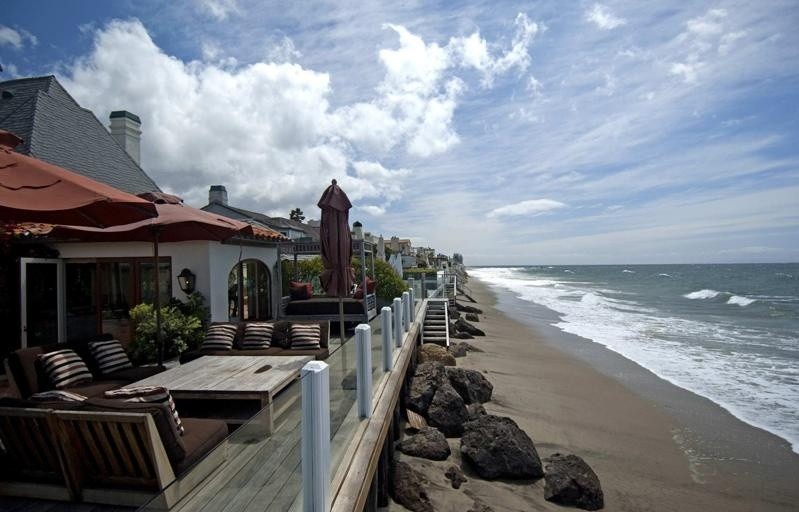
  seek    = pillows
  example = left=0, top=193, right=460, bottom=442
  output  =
left=286, top=322, right=321, bottom=350
left=200, top=325, right=238, bottom=350
left=104, top=385, right=184, bottom=435
left=353, top=276, right=377, bottom=298
left=29, top=390, right=87, bottom=401
left=88, top=340, right=132, bottom=374
left=34, top=349, right=92, bottom=388
left=242, top=322, right=275, bottom=349
left=288, top=281, right=311, bottom=300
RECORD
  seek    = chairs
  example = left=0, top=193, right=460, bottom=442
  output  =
left=3, top=340, right=126, bottom=398
left=80, top=334, right=164, bottom=382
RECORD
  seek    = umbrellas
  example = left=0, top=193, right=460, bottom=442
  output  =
left=375, top=234, right=386, bottom=262
left=1, top=190, right=281, bottom=367
left=1, top=129, right=160, bottom=229
left=316, top=180, right=353, bottom=345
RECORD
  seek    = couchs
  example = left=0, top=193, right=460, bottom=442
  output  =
left=0, top=400, right=228, bottom=512
left=280, top=294, right=377, bottom=325
left=180, top=320, right=331, bottom=364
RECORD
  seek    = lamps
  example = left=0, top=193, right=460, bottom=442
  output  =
left=177, top=268, right=196, bottom=295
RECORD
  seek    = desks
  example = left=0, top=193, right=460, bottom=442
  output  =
left=121, top=356, right=315, bottom=433
